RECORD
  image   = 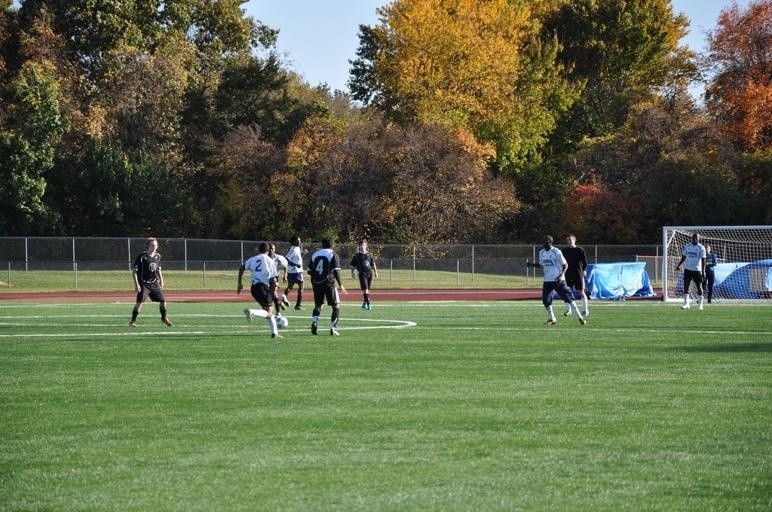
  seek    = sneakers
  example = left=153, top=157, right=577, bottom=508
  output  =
left=362, top=305, right=368, bottom=310
left=275, top=294, right=305, bottom=318
left=272, top=333, right=283, bottom=339
left=681, top=304, right=691, bottom=309
left=698, top=304, right=703, bottom=310
left=161, top=317, right=172, bottom=326
left=547, top=310, right=590, bottom=326
left=330, top=328, right=339, bottom=336
left=311, top=322, right=318, bottom=335
left=244, top=308, right=252, bottom=323
left=129, top=321, right=136, bottom=327
left=367, top=305, right=371, bottom=310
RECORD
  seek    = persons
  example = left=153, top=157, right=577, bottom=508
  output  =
left=558, top=234, right=590, bottom=316
left=280, top=235, right=308, bottom=310
left=129, top=237, right=174, bottom=328
left=306, top=234, right=348, bottom=335
left=525, top=233, right=586, bottom=327
left=268, top=242, right=289, bottom=315
left=675, top=233, right=706, bottom=310
left=696, top=244, right=717, bottom=304
left=236, top=241, right=283, bottom=337
left=349, top=237, right=378, bottom=310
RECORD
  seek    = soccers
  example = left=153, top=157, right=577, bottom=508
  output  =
left=275, top=316, right=288, bottom=329
